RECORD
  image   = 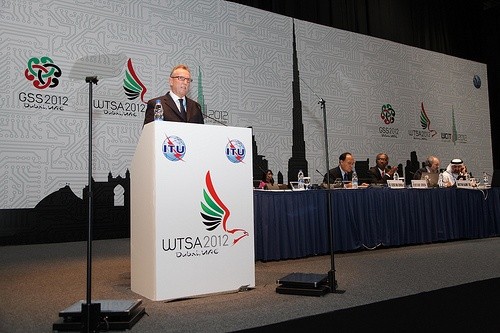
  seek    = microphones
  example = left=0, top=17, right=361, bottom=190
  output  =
left=196, top=104, right=227, bottom=126
left=316, top=169, right=333, bottom=189
left=258, top=166, right=279, bottom=190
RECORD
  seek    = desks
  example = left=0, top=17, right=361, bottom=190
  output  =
left=253, top=185, right=500, bottom=262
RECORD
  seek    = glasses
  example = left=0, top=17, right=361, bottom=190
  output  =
left=171, top=76, right=193, bottom=83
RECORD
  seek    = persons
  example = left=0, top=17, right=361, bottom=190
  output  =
left=412, top=156, right=440, bottom=184
left=324, top=152, right=369, bottom=188
left=259, top=170, right=274, bottom=189
left=142, top=64, right=205, bottom=130
left=438, top=159, right=470, bottom=188
left=368, top=153, right=397, bottom=183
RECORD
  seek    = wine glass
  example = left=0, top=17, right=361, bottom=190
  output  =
left=303, top=177, right=311, bottom=190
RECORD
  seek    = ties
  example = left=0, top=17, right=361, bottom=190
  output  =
left=344, top=172, right=347, bottom=181
left=382, top=172, right=384, bottom=178
left=178, top=99, right=186, bottom=122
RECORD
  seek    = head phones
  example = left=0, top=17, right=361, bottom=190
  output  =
left=427, top=161, right=431, bottom=167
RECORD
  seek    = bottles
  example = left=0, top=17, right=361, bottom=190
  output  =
left=483, top=172, right=488, bottom=184
left=154, top=100, right=164, bottom=120
left=298, top=169, right=304, bottom=189
left=352, top=172, right=358, bottom=189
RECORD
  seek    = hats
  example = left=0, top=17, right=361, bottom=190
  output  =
left=446, top=159, right=465, bottom=175
